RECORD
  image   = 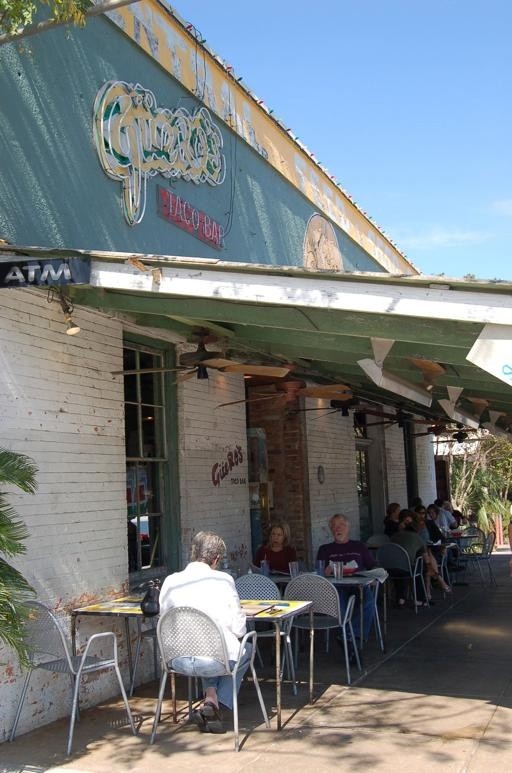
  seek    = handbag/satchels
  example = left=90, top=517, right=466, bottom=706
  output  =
left=141, top=579, right=161, bottom=617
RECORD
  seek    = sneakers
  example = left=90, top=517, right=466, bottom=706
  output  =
left=193, top=701, right=226, bottom=732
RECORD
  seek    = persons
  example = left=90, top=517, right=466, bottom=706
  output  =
left=158, top=530, right=255, bottom=735
left=250, top=521, right=298, bottom=666
left=383, top=497, right=464, bottom=611
left=315, top=514, right=376, bottom=667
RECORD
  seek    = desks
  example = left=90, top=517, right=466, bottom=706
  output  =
left=256, top=571, right=387, bottom=652
left=69, top=593, right=315, bottom=732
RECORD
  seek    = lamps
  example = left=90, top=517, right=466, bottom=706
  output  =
left=46, top=286, right=80, bottom=334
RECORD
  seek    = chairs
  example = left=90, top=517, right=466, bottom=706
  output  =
left=149, top=606, right=271, bottom=751
left=366, top=533, right=396, bottom=603
left=233, top=573, right=297, bottom=695
left=375, top=542, right=431, bottom=614
left=388, top=520, right=487, bottom=601
left=461, top=531, right=496, bottom=585
left=282, top=573, right=362, bottom=684
left=8, top=600, right=138, bottom=756
left=129, top=615, right=160, bottom=698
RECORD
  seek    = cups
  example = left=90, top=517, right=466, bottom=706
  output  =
left=259, top=560, right=271, bottom=570
left=333, top=561, right=343, bottom=580
left=288, top=561, right=299, bottom=579
left=314, top=560, right=327, bottom=576
left=297, top=559, right=304, bottom=573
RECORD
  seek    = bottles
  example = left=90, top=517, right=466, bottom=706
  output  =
left=248, top=566, right=252, bottom=574
left=221, top=554, right=229, bottom=570
left=447, top=529, right=450, bottom=537
left=441, top=524, right=447, bottom=537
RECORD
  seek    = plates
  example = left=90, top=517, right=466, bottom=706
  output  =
left=342, top=568, right=361, bottom=574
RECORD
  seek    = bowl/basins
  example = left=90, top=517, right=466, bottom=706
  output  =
left=451, top=532, right=463, bottom=536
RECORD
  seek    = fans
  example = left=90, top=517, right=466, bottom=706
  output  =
left=432, top=422, right=493, bottom=445
left=406, top=417, right=481, bottom=439
left=110, top=327, right=289, bottom=384
left=212, top=365, right=353, bottom=414
left=352, top=403, right=445, bottom=435
left=294, top=393, right=386, bottom=421
